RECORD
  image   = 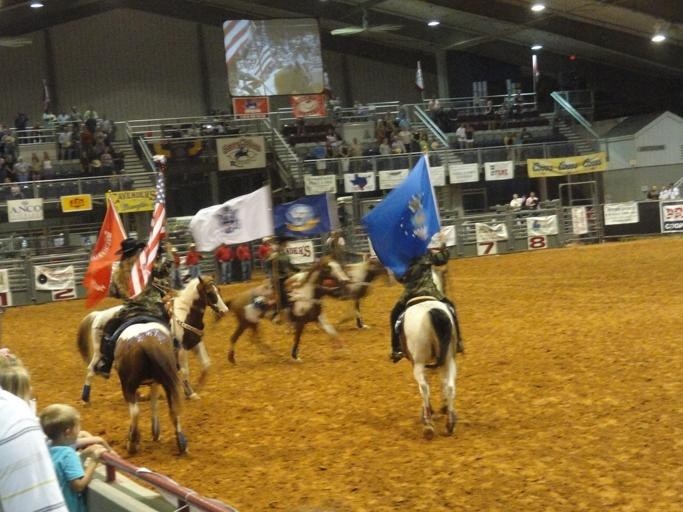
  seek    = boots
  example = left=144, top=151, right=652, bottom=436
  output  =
left=90, top=334, right=116, bottom=380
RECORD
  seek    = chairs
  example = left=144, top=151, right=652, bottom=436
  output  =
left=281, top=108, right=580, bottom=176
left=22, top=168, right=134, bottom=199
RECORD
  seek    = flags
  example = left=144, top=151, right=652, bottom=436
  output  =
left=270, top=191, right=332, bottom=237
left=188, top=184, right=273, bottom=253
left=131, top=169, right=167, bottom=298
left=359, top=155, right=440, bottom=280
left=83, top=199, right=125, bottom=310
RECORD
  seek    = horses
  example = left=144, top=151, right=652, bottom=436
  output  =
left=75, top=273, right=230, bottom=407
left=111, top=317, right=191, bottom=458
left=211, top=252, right=351, bottom=365
left=397, top=248, right=461, bottom=441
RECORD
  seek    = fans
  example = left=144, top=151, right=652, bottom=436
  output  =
left=331, top=8, right=404, bottom=45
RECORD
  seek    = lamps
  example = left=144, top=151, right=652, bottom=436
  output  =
left=651, top=22, right=667, bottom=42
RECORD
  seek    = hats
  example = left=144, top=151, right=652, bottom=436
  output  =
left=113, top=238, right=146, bottom=257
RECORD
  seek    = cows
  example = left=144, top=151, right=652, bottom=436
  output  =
left=283, top=255, right=390, bottom=329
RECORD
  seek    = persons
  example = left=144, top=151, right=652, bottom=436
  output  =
left=38, top=403, right=109, bottom=512
left=0, top=104, right=125, bottom=199
left=326, top=108, right=441, bottom=174
left=647, top=183, right=680, bottom=199
left=510, top=193, right=526, bottom=210
left=390, top=230, right=464, bottom=362
left=97, top=231, right=345, bottom=378
left=0, top=366, right=34, bottom=402
left=525, top=192, right=539, bottom=209
left=0, top=385, right=68, bottom=512
left=447, top=99, right=533, bottom=149
